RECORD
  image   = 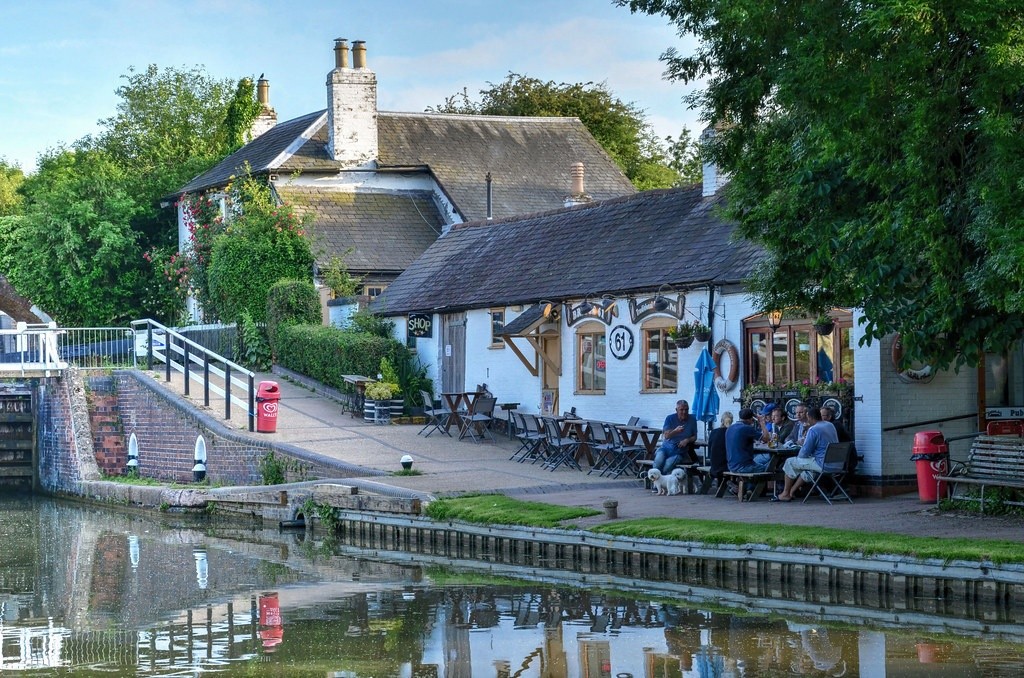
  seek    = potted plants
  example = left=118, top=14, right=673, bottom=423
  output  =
left=364, top=357, right=405, bottom=424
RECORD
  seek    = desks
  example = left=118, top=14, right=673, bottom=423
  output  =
left=565, top=419, right=626, bottom=470
left=749, top=445, right=801, bottom=502
left=340, top=374, right=374, bottom=420
left=440, top=392, right=486, bottom=439
left=615, top=425, right=661, bottom=471
left=657, top=438, right=708, bottom=483
left=530, top=414, right=576, bottom=460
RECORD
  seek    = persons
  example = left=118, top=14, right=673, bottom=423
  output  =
left=765, top=402, right=813, bottom=496
left=768, top=406, right=845, bottom=502
left=727, top=402, right=794, bottom=495
left=651, top=400, right=698, bottom=493
left=798, top=406, right=859, bottom=497
left=706, top=412, right=733, bottom=493
left=724, top=409, right=767, bottom=501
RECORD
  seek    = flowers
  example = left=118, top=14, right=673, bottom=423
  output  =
left=810, top=312, right=840, bottom=326
left=691, top=320, right=711, bottom=332
left=740, top=377, right=850, bottom=390
left=668, top=320, right=694, bottom=340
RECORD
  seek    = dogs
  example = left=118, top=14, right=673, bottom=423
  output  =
left=647, top=468, right=693, bottom=496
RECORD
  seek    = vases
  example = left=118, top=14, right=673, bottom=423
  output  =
left=694, top=332, right=711, bottom=342
left=743, top=390, right=846, bottom=399
left=674, top=335, right=695, bottom=350
left=813, top=325, right=836, bottom=335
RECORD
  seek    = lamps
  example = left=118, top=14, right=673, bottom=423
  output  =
left=550, top=310, right=560, bottom=320
left=766, top=306, right=785, bottom=332
left=601, top=294, right=632, bottom=312
left=578, top=292, right=601, bottom=314
left=654, top=283, right=688, bottom=310
left=538, top=300, right=571, bottom=317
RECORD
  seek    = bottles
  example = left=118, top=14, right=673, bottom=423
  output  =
left=768, top=433, right=777, bottom=448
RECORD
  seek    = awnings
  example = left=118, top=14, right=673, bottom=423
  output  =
left=494, top=299, right=561, bottom=377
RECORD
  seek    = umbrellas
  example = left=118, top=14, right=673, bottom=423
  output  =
left=688, top=346, right=720, bottom=467
left=815, top=346, right=833, bottom=383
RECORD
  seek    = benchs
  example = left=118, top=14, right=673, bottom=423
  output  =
left=934, top=434, right=1024, bottom=518
left=636, top=459, right=700, bottom=497
left=695, top=465, right=774, bottom=503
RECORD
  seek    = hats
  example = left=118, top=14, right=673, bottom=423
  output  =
left=759, top=403, right=779, bottom=416
left=739, top=409, right=754, bottom=419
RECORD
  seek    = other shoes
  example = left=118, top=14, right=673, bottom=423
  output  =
left=725, top=480, right=830, bottom=503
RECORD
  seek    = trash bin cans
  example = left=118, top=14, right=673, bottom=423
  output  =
left=256, top=380, right=281, bottom=434
left=259, top=593, right=284, bottom=652
left=916, top=636, right=937, bottom=661
left=911, top=429, right=949, bottom=505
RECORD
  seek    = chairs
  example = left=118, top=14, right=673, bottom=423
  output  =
left=802, top=440, right=854, bottom=505
left=415, top=390, right=452, bottom=438
left=509, top=411, right=647, bottom=480
left=459, top=397, right=498, bottom=443
